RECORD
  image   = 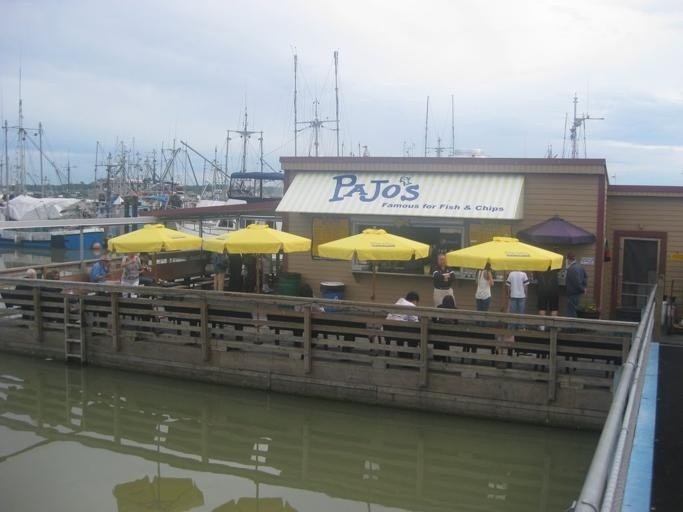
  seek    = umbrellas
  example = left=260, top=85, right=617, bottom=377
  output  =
left=318, top=229, right=430, bottom=325
left=108, top=224, right=203, bottom=281
left=202, top=224, right=311, bottom=339
left=517, top=214, right=596, bottom=246
left=446, top=237, right=564, bottom=311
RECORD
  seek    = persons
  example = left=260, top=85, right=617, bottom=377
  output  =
left=213, top=249, right=272, bottom=297
left=16, top=266, right=62, bottom=322
left=565, top=253, right=588, bottom=333
left=533, top=267, right=559, bottom=331
left=475, top=263, right=494, bottom=326
left=506, top=271, right=530, bottom=330
left=382, top=291, right=421, bottom=368
left=432, top=254, right=455, bottom=320
left=89, top=252, right=153, bottom=298
left=433, top=296, right=458, bottom=362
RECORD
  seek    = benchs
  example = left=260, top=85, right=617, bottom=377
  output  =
left=0, top=284, right=633, bottom=378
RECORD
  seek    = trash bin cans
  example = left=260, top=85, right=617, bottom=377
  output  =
left=320, top=281, right=345, bottom=312
left=278, top=271, right=301, bottom=296
